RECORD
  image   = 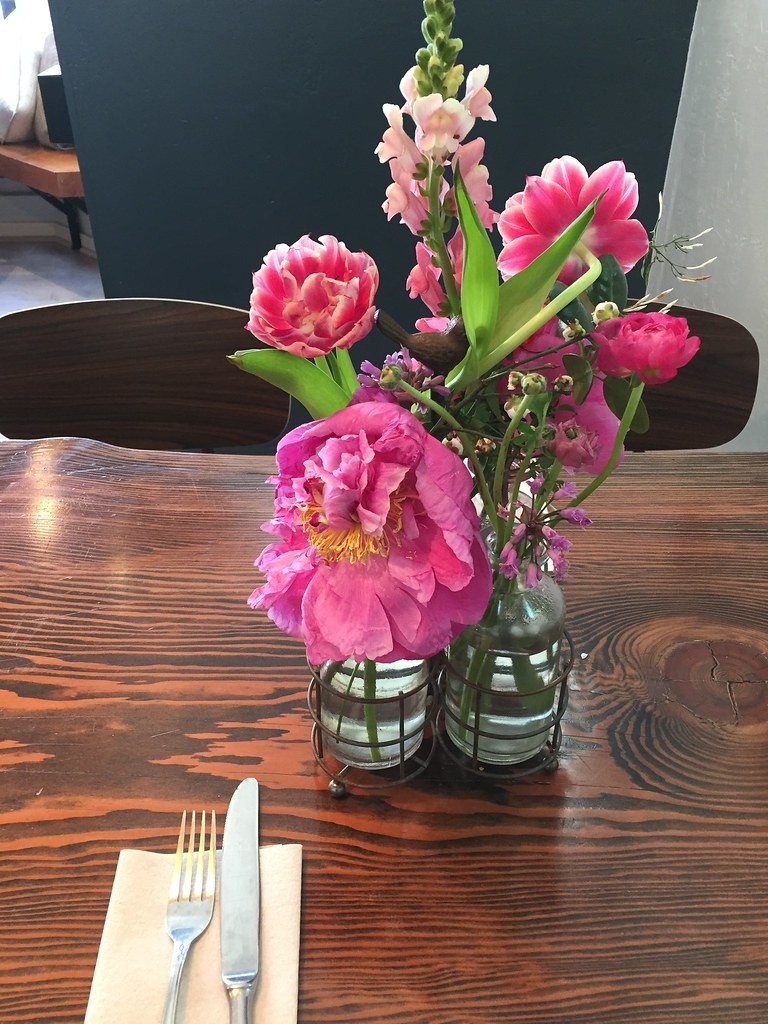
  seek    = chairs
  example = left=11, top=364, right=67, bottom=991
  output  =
left=0, top=299, right=296, bottom=455
left=620, top=290, right=762, bottom=454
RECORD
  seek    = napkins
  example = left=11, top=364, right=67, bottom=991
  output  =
left=82, top=836, right=304, bottom=1024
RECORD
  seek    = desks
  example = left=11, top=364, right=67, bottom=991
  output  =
left=0, top=434, right=768, bottom=1024
left=0, top=140, right=96, bottom=252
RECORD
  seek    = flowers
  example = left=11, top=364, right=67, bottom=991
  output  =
left=445, top=524, right=578, bottom=769
left=227, top=1, right=702, bottom=669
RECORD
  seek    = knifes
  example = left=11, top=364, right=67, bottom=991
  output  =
left=219, top=777, right=262, bottom=1024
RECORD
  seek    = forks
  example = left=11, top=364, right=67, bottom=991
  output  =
left=161, top=809, right=216, bottom=1024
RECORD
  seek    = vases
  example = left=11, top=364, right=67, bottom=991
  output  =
left=319, top=650, right=433, bottom=772
